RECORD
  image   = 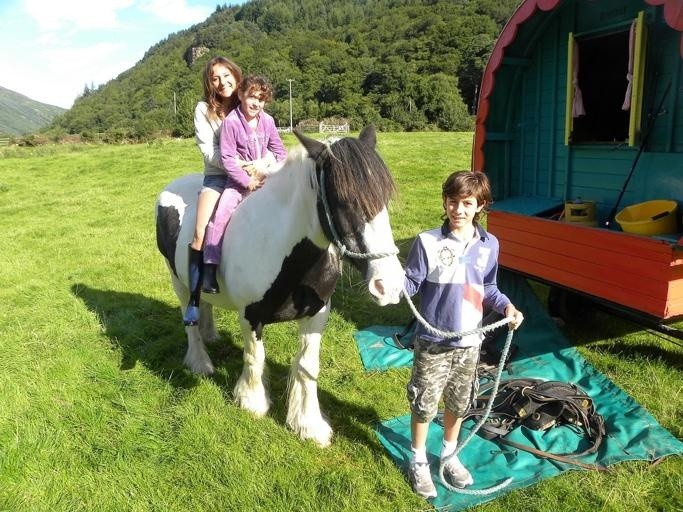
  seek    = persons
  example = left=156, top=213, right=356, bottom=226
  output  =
left=398, top=168, right=525, bottom=498
left=181, top=57, right=277, bottom=326
left=201, top=73, right=288, bottom=295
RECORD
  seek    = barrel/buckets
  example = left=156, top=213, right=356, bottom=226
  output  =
left=615, top=198, right=678, bottom=236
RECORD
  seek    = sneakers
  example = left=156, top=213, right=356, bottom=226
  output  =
left=438, top=455, right=474, bottom=490
left=408, top=462, right=438, bottom=499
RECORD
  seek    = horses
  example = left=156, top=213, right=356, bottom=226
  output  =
left=156, top=123, right=408, bottom=449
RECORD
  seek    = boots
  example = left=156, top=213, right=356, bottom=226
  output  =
left=202, top=262, right=221, bottom=294
left=182, top=244, right=204, bottom=327
